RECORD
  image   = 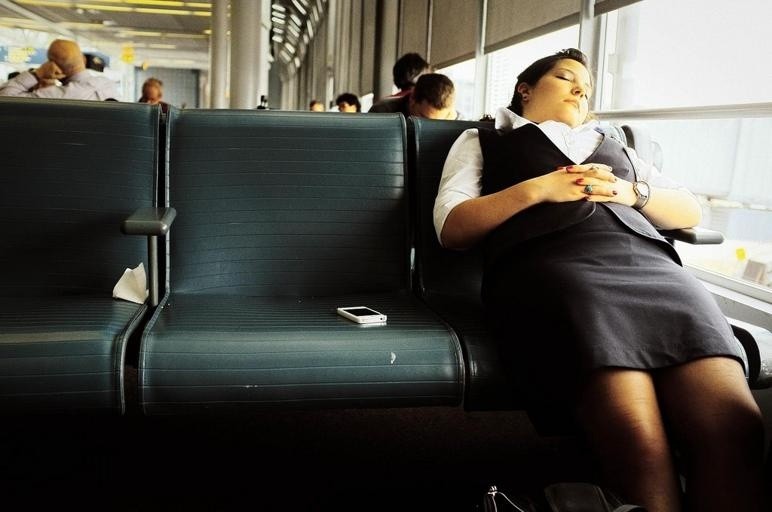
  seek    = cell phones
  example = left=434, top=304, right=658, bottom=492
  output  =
left=338, top=306, right=387, bottom=324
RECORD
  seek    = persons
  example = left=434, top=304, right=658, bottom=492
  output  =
left=336, top=92, right=362, bottom=114
left=386, top=51, right=430, bottom=96
left=366, top=72, right=470, bottom=123
left=0, top=39, right=123, bottom=102
left=309, top=100, right=326, bottom=112
left=138, top=77, right=175, bottom=114
left=8, top=53, right=105, bottom=93
left=430, top=48, right=771, bottom=510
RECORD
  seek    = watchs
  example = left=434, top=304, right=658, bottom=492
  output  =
left=631, top=179, right=651, bottom=210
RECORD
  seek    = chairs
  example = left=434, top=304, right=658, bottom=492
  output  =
left=139, top=107, right=468, bottom=419
left=406, top=114, right=772, bottom=418
left=0, top=95, right=163, bottom=418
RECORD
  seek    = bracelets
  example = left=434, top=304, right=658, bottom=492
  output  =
left=31, top=70, right=41, bottom=82
